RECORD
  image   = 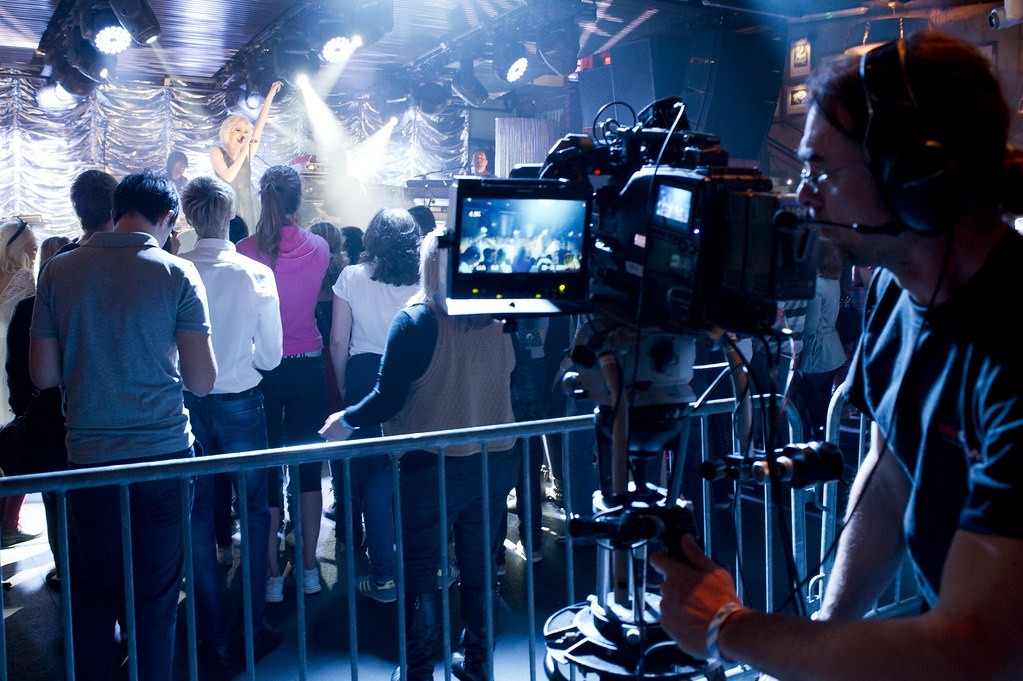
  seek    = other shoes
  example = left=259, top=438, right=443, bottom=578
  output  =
left=546, top=482, right=562, bottom=506
left=46, top=571, right=63, bottom=588
left=517, top=539, right=541, bottom=562
left=216, top=545, right=234, bottom=565
left=0, top=523, right=46, bottom=548
left=451, top=651, right=470, bottom=681
left=507, top=487, right=517, bottom=513
left=495, top=565, right=506, bottom=576
left=593, top=491, right=625, bottom=513
left=554, top=535, right=598, bottom=557
left=118, top=638, right=128, bottom=668
left=323, top=503, right=335, bottom=521
left=713, top=494, right=732, bottom=508
left=391, top=664, right=407, bottom=681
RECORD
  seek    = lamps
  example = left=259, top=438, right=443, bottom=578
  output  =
left=384, top=0, right=597, bottom=118
left=212, top=0, right=394, bottom=114
left=34, top=0, right=160, bottom=109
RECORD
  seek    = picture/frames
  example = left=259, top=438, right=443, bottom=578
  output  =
left=781, top=33, right=815, bottom=118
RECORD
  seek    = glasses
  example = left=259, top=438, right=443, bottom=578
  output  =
left=801, top=153, right=871, bottom=194
left=0, top=216, right=27, bottom=246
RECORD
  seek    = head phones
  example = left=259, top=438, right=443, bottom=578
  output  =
left=859, top=38, right=978, bottom=239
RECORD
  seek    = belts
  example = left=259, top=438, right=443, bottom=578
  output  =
left=283, top=349, right=321, bottom=359
left=183, top=387, right=259, bottom=401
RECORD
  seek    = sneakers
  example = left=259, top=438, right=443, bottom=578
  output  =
left=265, top=575, right=283, bottom=602
left=435, top=565, right=459, bottom=589
left=293, top=566, right=321, bottom=594
left=355, top=575, right=397, bottom=602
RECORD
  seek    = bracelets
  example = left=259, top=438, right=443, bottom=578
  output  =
left=705, top=602, right=745, bottom=669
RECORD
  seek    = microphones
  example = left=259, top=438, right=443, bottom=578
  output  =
left=771, top=210, right=889, bottom=237
left=463, top=161, right=469, bottom=169
left=240, top=136, right=258, bottom=143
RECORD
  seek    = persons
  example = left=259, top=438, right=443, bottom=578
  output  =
left=495, top=232, right=871, bottom=587
left=647, top=27, right=1023, bottom=681
left=28, top=173, right=217, bottom=681
left=0, top=164, right=436, bottom=681
left=318, top=224, right=518, bottom=681
left=460, top=226, right=581, bottom=273
left=465, top=149, right=499, bottom=179
left=164, top=151, right=192, bottom=232
left=210, top=81, right=284, bottom=236
